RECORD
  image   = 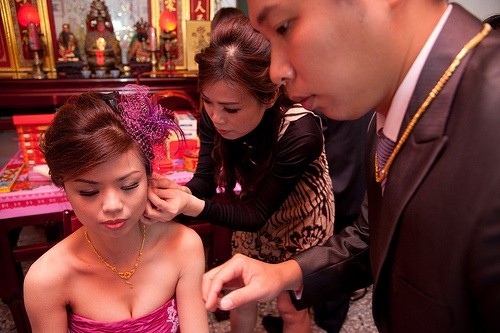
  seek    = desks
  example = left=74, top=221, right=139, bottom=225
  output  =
left=0, top=111, right=244, bottom=333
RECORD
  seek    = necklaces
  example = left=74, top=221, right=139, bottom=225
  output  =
left=83, top=224, right=145, bottom=288
left=374, top=22, right=491, bottom=182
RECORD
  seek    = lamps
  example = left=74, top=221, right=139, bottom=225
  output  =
left=16, top=5, right=46, bottom=79
left=156, top=12, right=178, bottom=69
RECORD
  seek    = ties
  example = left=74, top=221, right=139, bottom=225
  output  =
left=376, top=132, right=396, bottom=196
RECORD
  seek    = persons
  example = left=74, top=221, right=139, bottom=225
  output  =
left=129, top=23, right=151, bottom=62
left=142, top=15, right=334, bottom=333
left=86, top=0, right=115, bottom=46
left=201, top=0, right=500, bottom=333
left=24, top=93, right=209, bottom=333
left=57, top=24, right=76, bottom=57
